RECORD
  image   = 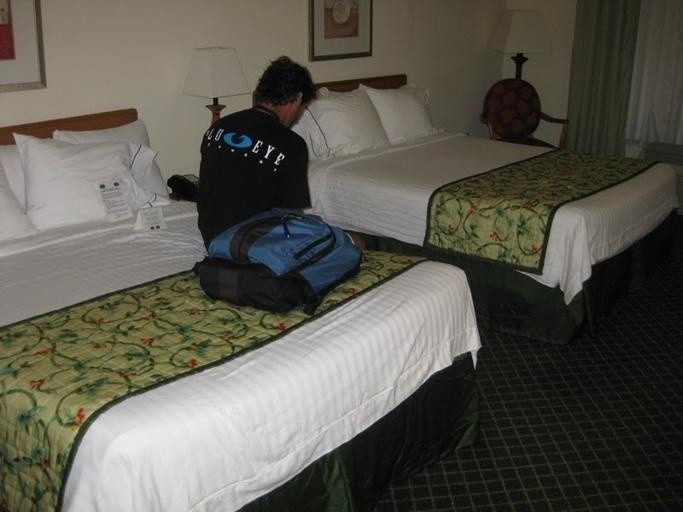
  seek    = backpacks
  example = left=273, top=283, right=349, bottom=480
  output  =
left=197, top=210, right=363, bottom=312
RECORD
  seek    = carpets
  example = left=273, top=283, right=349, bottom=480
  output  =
left=237, top=207, right=683, bottom=512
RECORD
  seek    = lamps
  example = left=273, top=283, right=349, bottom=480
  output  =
left=492, top=9, right=547, bottom=78
left=183, top=48, right=252, bottom=121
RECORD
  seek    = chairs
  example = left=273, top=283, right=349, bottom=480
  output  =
left=481, top=78, right=568, bottom=148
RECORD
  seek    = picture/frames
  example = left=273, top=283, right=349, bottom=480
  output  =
left=308, top=0, right=373, bottom=62
left=0, top=0, right=47, bottom=92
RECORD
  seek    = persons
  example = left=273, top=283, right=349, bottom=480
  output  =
left=196, top=56, right=367, bottom=257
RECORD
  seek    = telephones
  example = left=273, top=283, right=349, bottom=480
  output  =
left=165, top=173, right=200, bottom=202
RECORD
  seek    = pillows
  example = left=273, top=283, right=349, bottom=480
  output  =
left=0, top=145, right=34, bottom=211
left=292, top=116, right=314, bottom=166
left=307, top=89, right=392, bottom=158
left=401, top=85, right=431, bottom=105
left=365, top=91, right=444, bottom=143
left=52, top=119, right=171, bottom=208
left=13, top=132, right=137, bottom=229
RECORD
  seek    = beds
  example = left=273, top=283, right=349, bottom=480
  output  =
left=291, top=74, right=683, bottom=337
left=0, top=108, right=482, bottom=512
